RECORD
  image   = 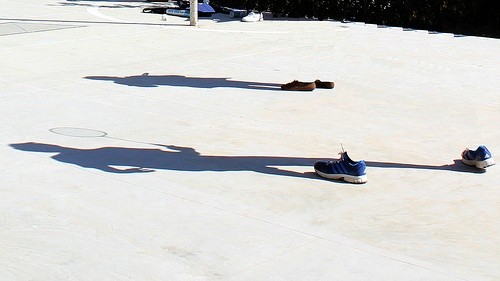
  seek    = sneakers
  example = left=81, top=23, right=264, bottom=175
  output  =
left=314, top=151, right=368, bottom=184
left=460, top=145, right=495, bottom=168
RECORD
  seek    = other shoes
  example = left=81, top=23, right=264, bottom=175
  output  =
left=281, top=79, right=316, bottom=90
left=314, top=79, right=334, bottom=88
left=241, top=12, right=264, bottom=21
